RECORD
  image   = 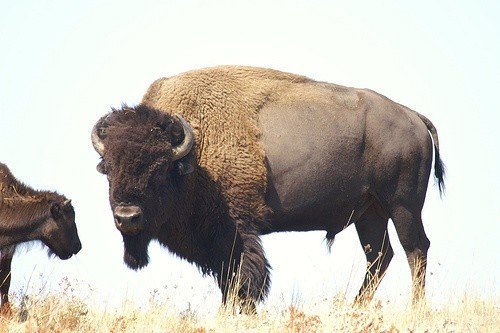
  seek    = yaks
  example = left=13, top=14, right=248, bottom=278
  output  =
left=89, top=63, right=446, bottom=315
left=0, top=161, right=83, bottom=320
